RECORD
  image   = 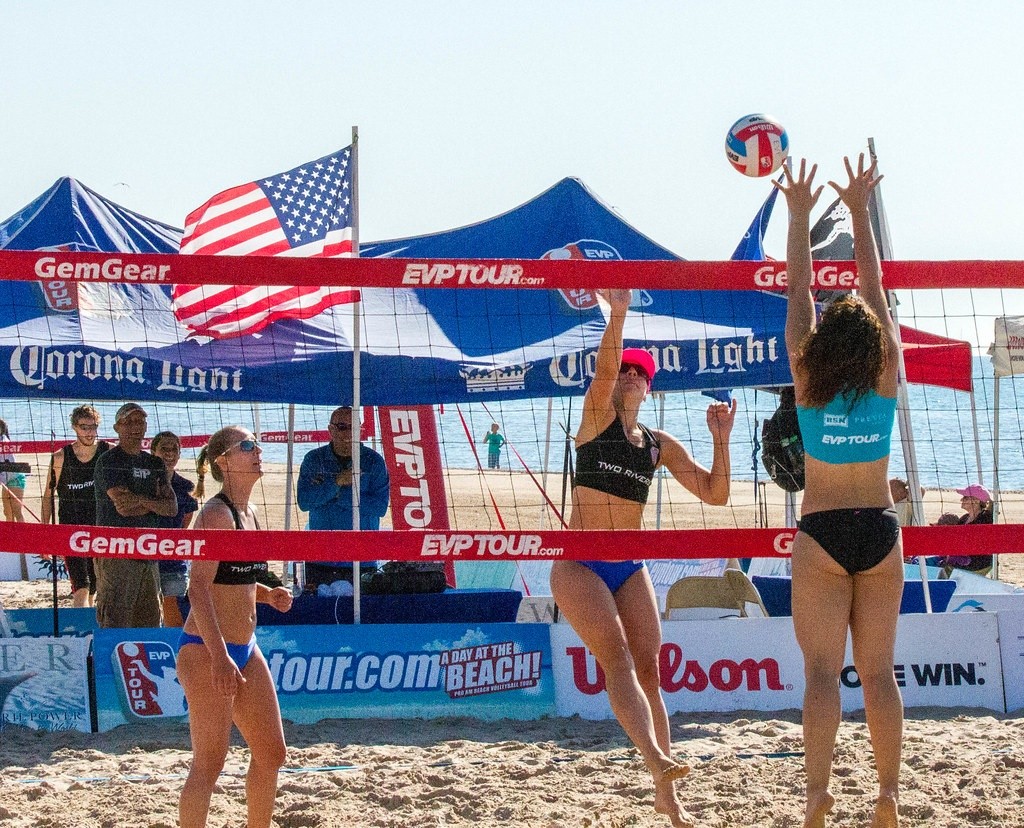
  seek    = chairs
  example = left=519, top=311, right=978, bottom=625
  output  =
left=665, top=567, right=769, bottom=621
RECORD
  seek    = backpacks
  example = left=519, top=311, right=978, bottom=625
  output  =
left=760, top=391, right=804, bottom=492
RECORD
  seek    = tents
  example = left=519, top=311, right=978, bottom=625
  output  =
left=0, top=176, right=829, bottom=625
left=889, top=324, right=983, bottom=486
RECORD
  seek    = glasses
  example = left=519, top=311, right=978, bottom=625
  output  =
left=330, top=421, right=352, bottom=431
left=214, top=438, right=261, bottom=463
left=75, top=422, right=99, bottom=431
left=620, top=362, right=650, bottom=380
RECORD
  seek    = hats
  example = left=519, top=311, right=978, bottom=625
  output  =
left=623, top=349, right=656, bottom=393
left=956, top=483, right=990, bottom=503
left=114, top=402, right=148, bottom=422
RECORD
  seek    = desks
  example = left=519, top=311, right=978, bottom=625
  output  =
left=752, top=575, right=956, bottom=616
left=177, top=586, right=523, bottom=627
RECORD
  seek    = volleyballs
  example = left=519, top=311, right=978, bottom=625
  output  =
left=725, top=113, right=790, bottom=178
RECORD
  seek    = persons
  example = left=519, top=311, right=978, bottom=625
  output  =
left=296, top=407, right=389, bottom=588
left=772, top=153, right=904, bottom=828
left=93, top=403, right=178, bottom=629
left=151, top=431, right=199, bottom=628
left=888, top=477, right=994, bottom=572
left=42, top=403, right=117, bottom=607
left=551, top=287, right=737, bottom=827
left=177, top=425, right=295, bottom=827
left=0, top=419, right=27, bottom=522
left=484, top=422, right=505, bottom=469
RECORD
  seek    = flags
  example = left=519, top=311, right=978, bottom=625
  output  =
left=171, top=144, right=361, bottom=342
